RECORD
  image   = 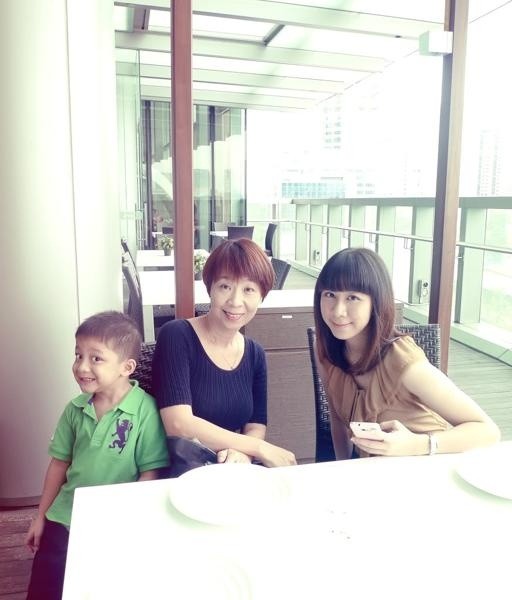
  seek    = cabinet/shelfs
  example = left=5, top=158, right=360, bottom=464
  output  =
left=219, top=289, right=405, bottom=460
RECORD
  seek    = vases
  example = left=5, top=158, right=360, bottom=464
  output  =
left=194, top=271, right=203, bottom=280
left=164, top=247, right=172, bottom=256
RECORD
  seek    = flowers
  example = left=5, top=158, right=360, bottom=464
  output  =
left=193, top=250, right=208, bottom=274
left=160, top=236, right=174, bottom=248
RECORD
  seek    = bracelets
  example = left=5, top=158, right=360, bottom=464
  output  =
left=426, top=431, right=437, bottom=456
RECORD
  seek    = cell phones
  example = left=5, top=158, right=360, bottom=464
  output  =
left=349, top=421, right=384, bottom=443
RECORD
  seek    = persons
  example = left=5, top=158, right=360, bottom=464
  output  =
left=312, top=248, right=502, bottom=462
left=26, top=312, right=170, bottom=600
left=150, top=237, right=298, bottom=480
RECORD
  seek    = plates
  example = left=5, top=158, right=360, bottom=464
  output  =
left=169, top=462, right=284, bottom=527
left=457, top=438, right=511, bottom=501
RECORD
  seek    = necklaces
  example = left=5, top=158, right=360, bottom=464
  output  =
left=226, top=354, right=240, bottom=371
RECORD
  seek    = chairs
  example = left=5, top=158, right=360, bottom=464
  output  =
left=226, top=226, right=254, bottom=242
left=213, top=221, right=236, bottom=231
left=162, top=226, right=173, bottom=233
left=263, top=222, right=278, bottom=256
left=128, top=340, right=157, bottom=398
left=307, top=323, right=441, bottom=463
left=121, top=236, right=144, bottom=328
left=271, top=258, right=291, bottom=289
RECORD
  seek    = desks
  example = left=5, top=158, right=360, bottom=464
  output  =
left=136, top=249, right=211, bottom=271
left=209, top=230, right=228, bottom=242
left=142, top=272, right=219, bottom=342
